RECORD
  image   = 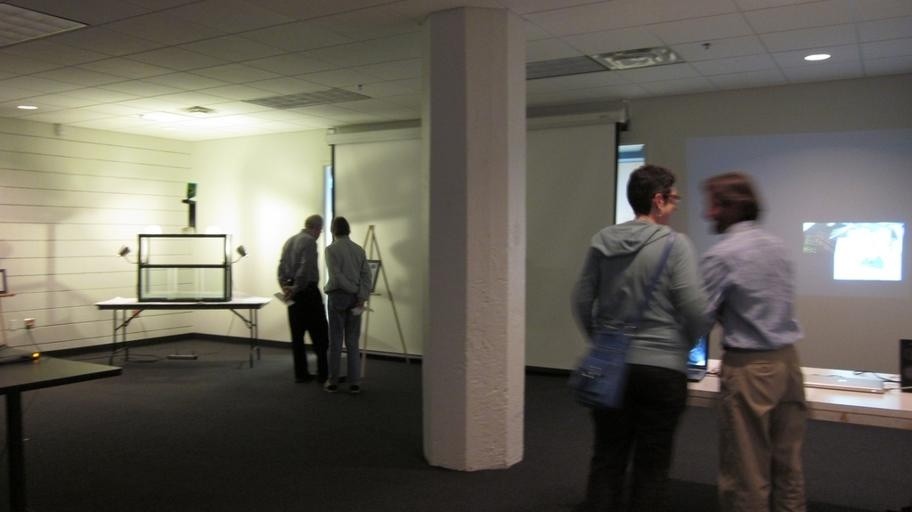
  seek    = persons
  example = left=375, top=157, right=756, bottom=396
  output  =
left=323, top=217, right=373, bottom=394
left=698, top=170, right=809, bottom=512
left=569, top=164, right=715, bottom=512
left=277, top=214, right=347, bottom=385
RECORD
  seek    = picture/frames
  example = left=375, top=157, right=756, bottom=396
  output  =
left=0, top=269, right=8, bottom=294
left=367, top=260, right=380, bottom=292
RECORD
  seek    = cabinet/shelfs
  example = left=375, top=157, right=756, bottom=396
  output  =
left=137, top=233, right=232, bottom=302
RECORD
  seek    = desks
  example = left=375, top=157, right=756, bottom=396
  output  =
left=93, top=297, right=272, bottom=368
left=0, top=357, right=123, bottom=512
left=669, top=358, right=912, bottom=512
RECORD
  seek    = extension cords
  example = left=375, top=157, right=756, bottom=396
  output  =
left=167, top=354, right=198, bottom=359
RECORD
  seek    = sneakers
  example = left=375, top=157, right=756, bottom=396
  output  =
left=297, top=373, right=359, bottom=393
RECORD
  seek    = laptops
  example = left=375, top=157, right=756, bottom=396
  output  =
left=804, top=373, right=886, bottom=394
left=687, top=334, right=709, bottom=382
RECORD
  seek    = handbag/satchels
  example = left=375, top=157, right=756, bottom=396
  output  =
left=568, top=333, right=628, bottom=408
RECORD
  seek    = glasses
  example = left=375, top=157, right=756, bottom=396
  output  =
left=661, top=192, right=680, bottom=204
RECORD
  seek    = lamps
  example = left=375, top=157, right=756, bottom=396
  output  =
left=232, top=245, right=248, bottom=264
left=119, top=246, right=137, bottom=265
